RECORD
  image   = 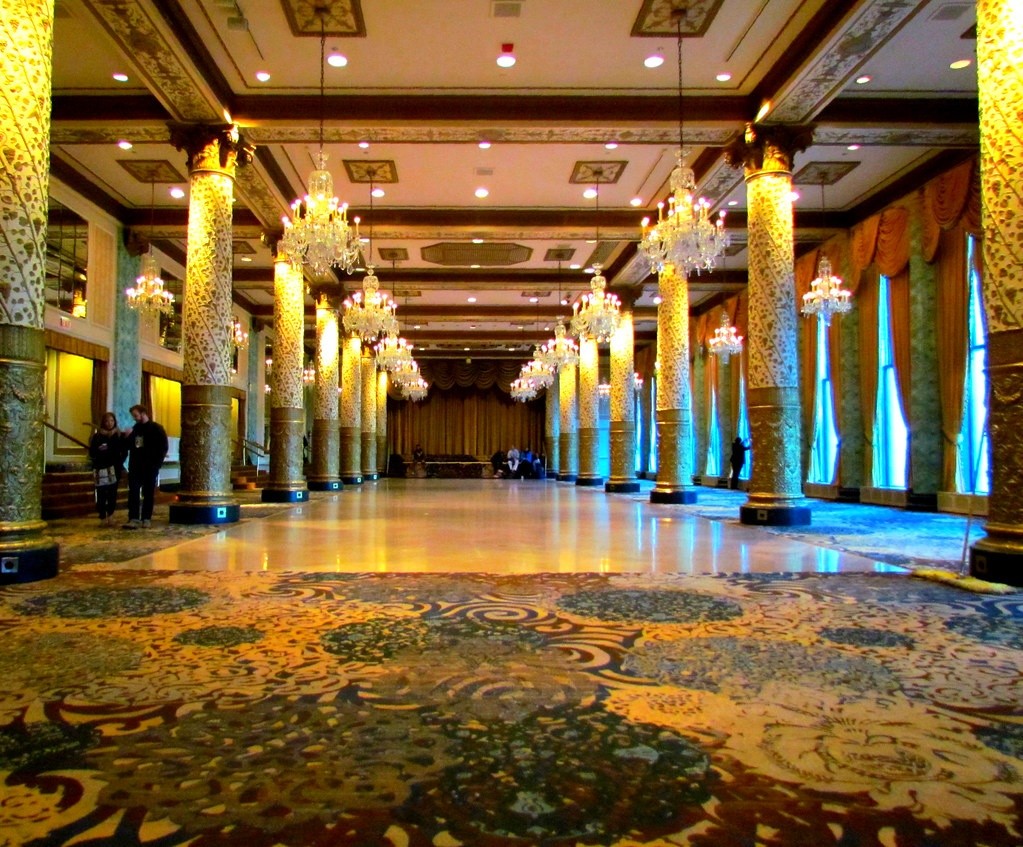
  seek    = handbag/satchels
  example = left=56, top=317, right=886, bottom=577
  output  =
left=93, top=449, right=117, bottom=486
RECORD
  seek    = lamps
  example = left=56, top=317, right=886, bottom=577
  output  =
left=339, top=168, right=398, bottom=345
left=278, top=3, right=365, bottom=277
left=302, top=361, right=317, bottom=384
left=374, top=256, right=433, bottom=403
left=234, top=324, right=249, bottom=348
left=509, top=254, right=581, bottom=402
left=640, top=7, right=730, bottom=278
left=802, top=174, right=852, bottom=325
left=128, top=169, right=176, bottom=324
left=569, top=171, right=624, bottom=342
left=708, top=251, right=744, bottom=364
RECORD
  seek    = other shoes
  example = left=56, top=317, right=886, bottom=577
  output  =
left=98, top=515, right=115, bottom=528
left=121, top=519, right=140, bottom=530
left=141, top=519, right=151, bottom=529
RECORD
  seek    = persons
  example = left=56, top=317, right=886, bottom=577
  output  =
left=413, top=444, right=425, bottom=464
left=89, top=412, right=128, bottom=529
left=730, top=436, right=751, bottom=488
left=506, top=446, right=543, bottom=479
left=122, top=405, right=168, bottom=529
